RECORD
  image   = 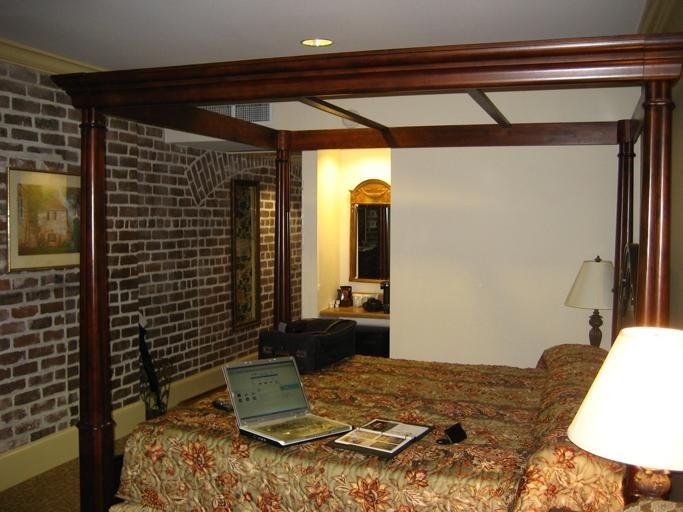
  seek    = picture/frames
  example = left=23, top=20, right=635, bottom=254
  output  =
left=7, top=167, right=81, bottom=273
left=231, top=178, right=260, bottom=331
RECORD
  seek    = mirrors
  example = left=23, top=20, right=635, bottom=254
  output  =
left=349, top=179, right=391, bottom=283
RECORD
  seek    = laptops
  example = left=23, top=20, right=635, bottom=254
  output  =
left=222, top=356, right=353, bottom=449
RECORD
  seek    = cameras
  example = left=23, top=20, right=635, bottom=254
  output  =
left=362, top=298, right=381, bottom=311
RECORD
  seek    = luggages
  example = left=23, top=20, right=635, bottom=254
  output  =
left=258, top=318, right=357, bottom=374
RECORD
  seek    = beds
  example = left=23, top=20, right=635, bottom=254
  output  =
left=115, top=242, right=639, bottom=512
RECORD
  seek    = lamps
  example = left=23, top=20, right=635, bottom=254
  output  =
left=564, top=256, right=614, bottom=348
left=564, top=327, right=683, bottom=511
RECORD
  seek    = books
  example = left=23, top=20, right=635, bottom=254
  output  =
left=324, top=416, right=434, bottom=460
